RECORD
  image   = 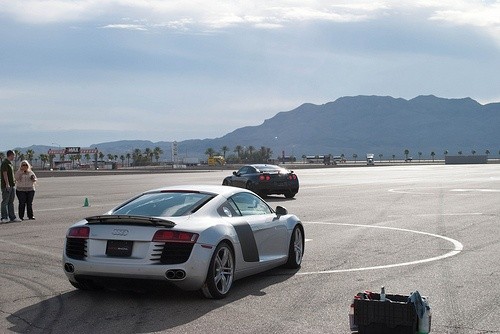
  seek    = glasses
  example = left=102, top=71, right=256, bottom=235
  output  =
left=22, top=164, right=28, bottom=166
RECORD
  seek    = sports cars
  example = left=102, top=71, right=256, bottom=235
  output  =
left=61, top=184, right=306, bottom=299
left=222, top=163, right=300, bottom=199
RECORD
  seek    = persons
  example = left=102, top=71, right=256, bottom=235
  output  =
left=0, top=150, right=22, bottom=223
left=14, top=161, right=37, bottom=220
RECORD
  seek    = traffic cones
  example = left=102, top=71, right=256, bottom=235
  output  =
left=83, top=196, right=90, bottom=207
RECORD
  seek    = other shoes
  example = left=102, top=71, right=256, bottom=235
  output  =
left=29, top=217, right=35, bottom=220
left=9, top=216, right=16, bottom=223
left=1, top=216, right=8, bottom=223
left=20, top=217, right=23, bottom=220
